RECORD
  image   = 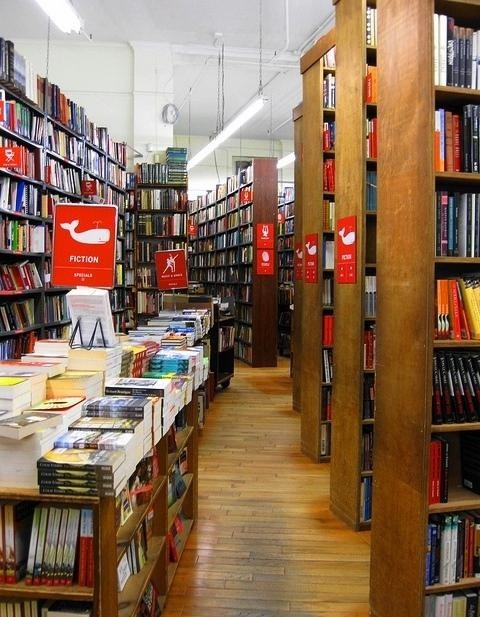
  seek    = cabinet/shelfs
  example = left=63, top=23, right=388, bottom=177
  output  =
left=0, top=324, right=199, bottom=616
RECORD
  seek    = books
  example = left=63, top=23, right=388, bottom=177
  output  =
left=234, top=305, right=253, bottom=363
left=322, top=51, right=337, bottom=456
left=424, top=12, right=480, bottom=616
left=186, top=166, right=254, bottom=303
left=360, top=8, right=379, bottom=528
left=1, top=308, right=214, bottom=617
left=2, top=37, right=126, bottom=360
left=276, top=187, right=296, bottom=359
left=125, top=231, right=188, bottom=314
left=125, top=148, right=189, bottom=237
